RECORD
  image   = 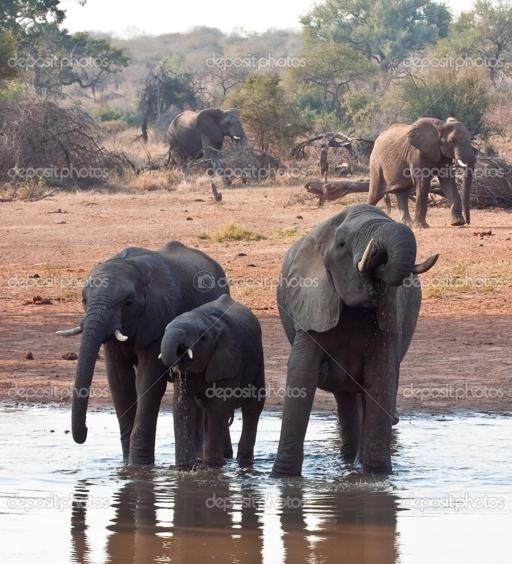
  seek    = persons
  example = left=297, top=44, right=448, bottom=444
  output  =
left=319, top=144, right=328, bottom=182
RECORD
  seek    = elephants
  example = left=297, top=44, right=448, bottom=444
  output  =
left=367, top=116, right=487, bottom=229
left=56, top=204, right=440, bottom=478
left=166, top=109, right=246, bottom=175
